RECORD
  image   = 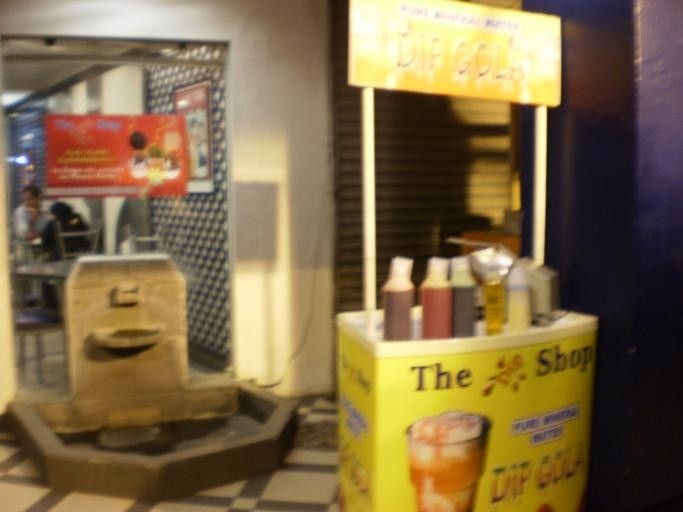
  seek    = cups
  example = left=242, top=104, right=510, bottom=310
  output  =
left=407, top=413, right=493, bottom=512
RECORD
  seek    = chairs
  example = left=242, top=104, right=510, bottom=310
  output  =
left=55, top=224, right=103, bottom=259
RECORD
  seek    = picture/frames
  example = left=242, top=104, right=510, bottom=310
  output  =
left=170, top=76, right=215, bottom=195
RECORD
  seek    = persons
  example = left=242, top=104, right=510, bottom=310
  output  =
left=13, top=187, right=52, bottom=240
left=42, top=202, right=88, bottom=261
left=127, top=131, right=150, bottom=178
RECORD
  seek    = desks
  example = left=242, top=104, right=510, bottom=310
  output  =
left=13, top=260, right=76, bottom=308
left=13, top=239, right=47, bottom=257
left=333, top=299, right=601, bottom=508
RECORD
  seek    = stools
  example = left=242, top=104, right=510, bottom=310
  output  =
left=14, top=310, right=67, bottom=386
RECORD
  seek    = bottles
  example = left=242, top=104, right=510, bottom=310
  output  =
left=381, top=257, right=415, bottom=340
left=452, top=256, right=477, bottom=337
left=484, top=264, right=504, bottom=336
left=507, top=267, right=531, bottom=334
left=421, top=256, right=452, bottom=339
